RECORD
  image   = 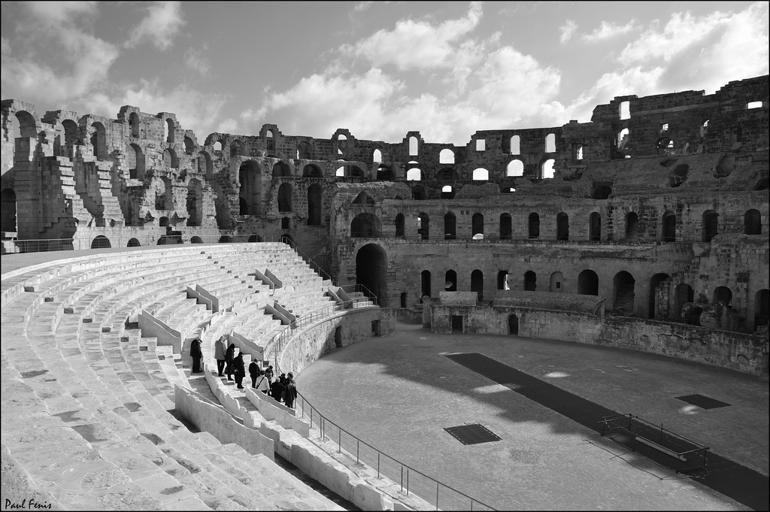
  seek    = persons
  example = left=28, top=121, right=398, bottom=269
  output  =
left=215, top=335, right=297, bottom=408
left=190, top=336, right=203, bottom=373
